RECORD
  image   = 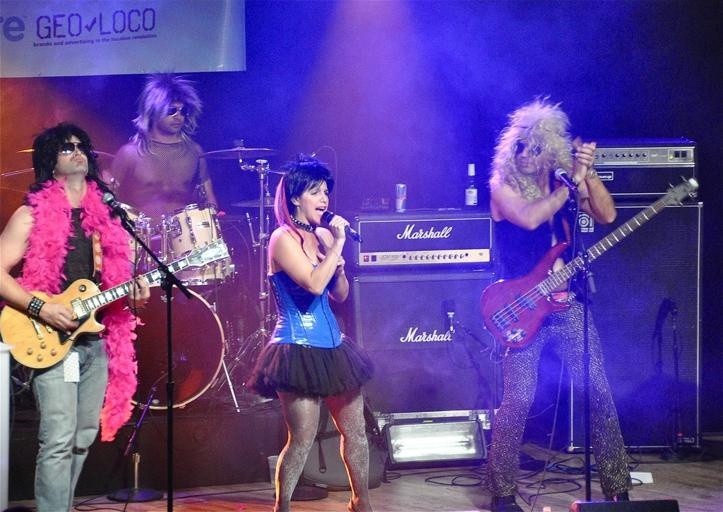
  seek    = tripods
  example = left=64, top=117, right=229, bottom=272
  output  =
left=466, top=356, right=548, bottom=474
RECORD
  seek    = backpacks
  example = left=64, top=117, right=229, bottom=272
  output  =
left=302, top=399, right=385, bottom=491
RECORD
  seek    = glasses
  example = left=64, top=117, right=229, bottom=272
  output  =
left=515, top=141, right=544, bottom=157
left=56, top=142, right=89, bottom=156
left=166, top=106, right=189, bottom=117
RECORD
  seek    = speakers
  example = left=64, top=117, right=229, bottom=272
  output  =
left=569, top=499, right=680, bottom=512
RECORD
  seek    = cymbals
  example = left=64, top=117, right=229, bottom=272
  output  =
left=16, top=148, right=116, bottom=159
left=200, top=148, right=278, bottom=160
left=233, top=198, right=278, bottom=208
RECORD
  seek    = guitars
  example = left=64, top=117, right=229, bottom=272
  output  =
left=480, top=177, right=699, bottom=348
left=0, top=239, right=229, bottom=370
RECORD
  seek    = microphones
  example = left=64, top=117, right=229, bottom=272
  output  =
left=554, top=167, right=577, bottom=193
left=321, top=210, right=363, bottom=243
left=446, top=299, right=456, bottom=334
left=101, top=192, right=135, bottom=227
left=670, top=306, right=678, bottom=333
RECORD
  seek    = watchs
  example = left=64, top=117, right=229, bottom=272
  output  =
left=584, top=170, right=597, bottom=179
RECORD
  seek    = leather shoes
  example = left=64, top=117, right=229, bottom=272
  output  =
left=488, top=495, right=526, bottom=512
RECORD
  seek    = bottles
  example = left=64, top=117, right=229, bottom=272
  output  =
left=464, top=163, right=478, bottom=210
left=394, top=182, right=406, bottom=214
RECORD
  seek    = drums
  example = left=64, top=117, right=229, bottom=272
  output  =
left=161, top=202, right=231, bottom=296
left=116, top=201, right=151, bottom=273
left=129, top=286, right=227, bottom=411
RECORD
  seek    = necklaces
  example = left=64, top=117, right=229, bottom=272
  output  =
left=290, top=215, right=316, bottom=233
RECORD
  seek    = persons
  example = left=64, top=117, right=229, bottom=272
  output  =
left=108, top=74, right=217, bottom=219
left=244, top=159, right=374, bottom=512
left=485, top=99, right=633, bottom=512
left=0, top=122, right=151, bottom=511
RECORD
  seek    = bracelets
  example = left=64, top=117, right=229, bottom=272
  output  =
left=26, top=295, right=45, bottom=318
